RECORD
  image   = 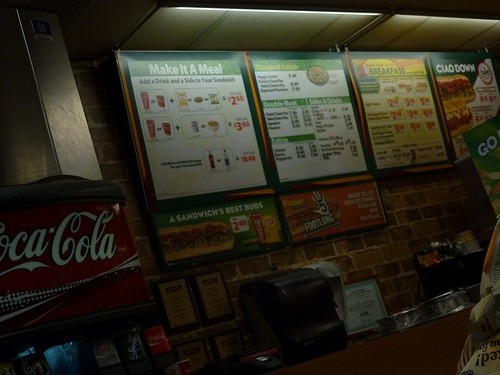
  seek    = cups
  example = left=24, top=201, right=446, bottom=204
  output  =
left=141, top=91, right=150, bottom=109
left=176, top=91, right=189, bottom=108
left=157, top=95, right=166, bottom=109
left=146, top=119, right=156, bottom=138
left=163, top=122, right=172, bottom=136
left=190, top=119, right=200, bottom=137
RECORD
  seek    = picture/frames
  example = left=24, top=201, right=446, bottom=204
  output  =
left=188, top=269, right=236, bottom=325
left=169, top=329, right=212, bottom=373
left=149, top=275, right=202, bottom=334
left=207, top=328, right=247, bottom=362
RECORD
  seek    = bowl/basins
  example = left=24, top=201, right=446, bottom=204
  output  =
left=453, top=230, right=480, bottom=251
left=207, top=120, right=219, bottom=132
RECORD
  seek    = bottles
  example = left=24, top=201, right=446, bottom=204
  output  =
left=208, top=150, right=215, bottom=169
left=223, top=150, right=230, bottom=167
